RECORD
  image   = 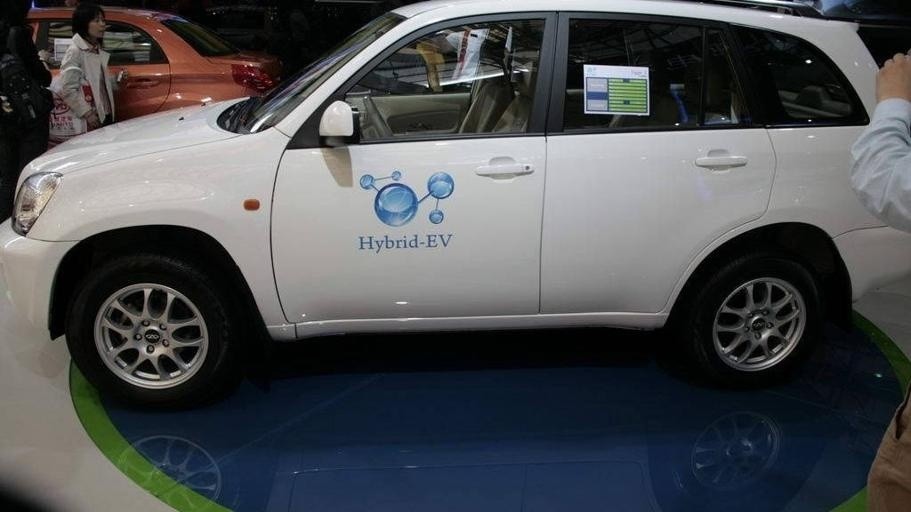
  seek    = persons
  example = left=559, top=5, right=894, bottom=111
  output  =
left=58, top=4, right=129, bottom=130
left=0, top=0, right=55, bottom=220
left=849, top=45, right=911, bottom=511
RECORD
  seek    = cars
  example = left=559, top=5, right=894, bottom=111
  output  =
left=195, top=7, right=288, bottom=66
left=25, top=7, right=284, bottom=155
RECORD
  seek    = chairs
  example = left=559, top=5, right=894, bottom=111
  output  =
left=488, top=40, right=538, bottom=136
left=457, top=41, right=516, bottom=136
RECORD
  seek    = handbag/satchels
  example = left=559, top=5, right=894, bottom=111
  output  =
left=46, top=86, right=87, bottom=142
left=1, top=51, right=53, bottom=123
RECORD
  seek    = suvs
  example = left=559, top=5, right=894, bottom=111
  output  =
left=69, top=333, right=900, bottom=512
left=1, top=0, right=911, bottom=411
